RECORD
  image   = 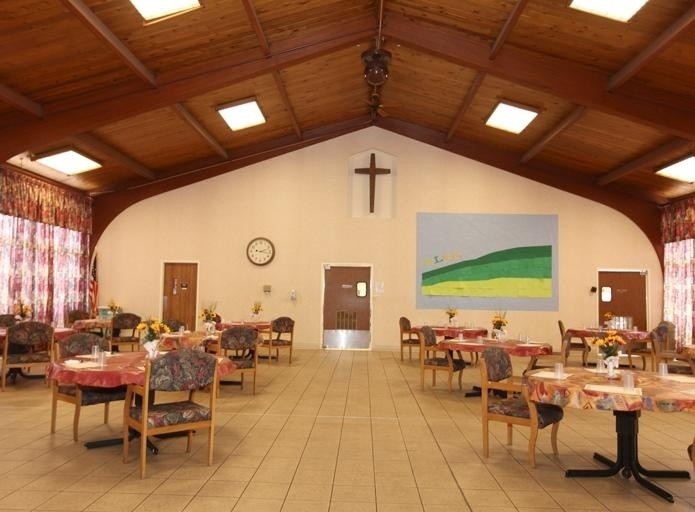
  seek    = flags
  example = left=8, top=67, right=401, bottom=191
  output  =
left=88, top=253, right=99, bottom=319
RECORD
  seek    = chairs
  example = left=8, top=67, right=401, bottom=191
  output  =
left=418, top=324, right=466, bottom=393
left=65, top=309, right=143, bottom=353
left=256, top=316, right=295, bottom=365
left=51, top=331, right=133, bottom=443
left=480, top=346, right=563, bottom=468
left=627, top=327, right=695, bottom=375
left=0, top=311, right=54, bottom=392
left=398, top=317, right=421, bottom=362
left=557, top=318, right=592, bottom=367
left=216, top=324, right=258, bottom=398
left=121, top=345, right=219, bottom=479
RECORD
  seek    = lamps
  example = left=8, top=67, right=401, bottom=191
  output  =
left=212, top=94, right=267, bottom=134
left=27, top=144, right=103, bottom=178
left=652, top=151, right=694, bottom=185
left=568, top=0, right=650, bottom=23
left=483, top=95, right=542, bottom=135
left=128, top=0, right=205, bottom=26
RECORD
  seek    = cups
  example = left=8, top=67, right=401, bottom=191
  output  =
left=658, top=361, right=669, bottom=377
left=597, top=356, right=607, bottom=372
left=620, top=370, right=634, bottom=392
left=476, top=335, right=483, bottom=344
left=91, top=344, right=99, bottom=358
left=458, top=334, right=463, bottom=342
left=98, top=351, right=108, bottom=367
left=606, top=362, right=617, bottom=380
left=178, top=325, right=185, bottom=335
left=553, top=361, right=564, bottom=380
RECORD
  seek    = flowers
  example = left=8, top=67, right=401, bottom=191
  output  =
left=445, top=306, right=458, bottom=320
left=247, top=301, right=264, bottom=315
left=590, top=329, right=626, bottom=357
left=11, top=302, right=33, bottom=316
left=491, top=309, right=511, bottom=331
left=138, top=315, right=170, bottom=342
left=199, top=306, right=217, bottom=322
left=105, top=302, right=121, bottom=315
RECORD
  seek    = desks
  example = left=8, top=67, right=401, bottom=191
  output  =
left=45, top=351, right=160, bottom=455
left=523, top=365, right=695, bottom=504
left=218, top=321, right=278, bottom=359
left=412, top=325, right=487, bottom=365
left=438, top=338, right=553, bottom=398
left=566, top=328, right=649, bottom=368
left=73, top=319, right=111, bottom=334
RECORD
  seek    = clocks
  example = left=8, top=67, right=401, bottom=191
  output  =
left=246, top=235, right=274, bottom=267
